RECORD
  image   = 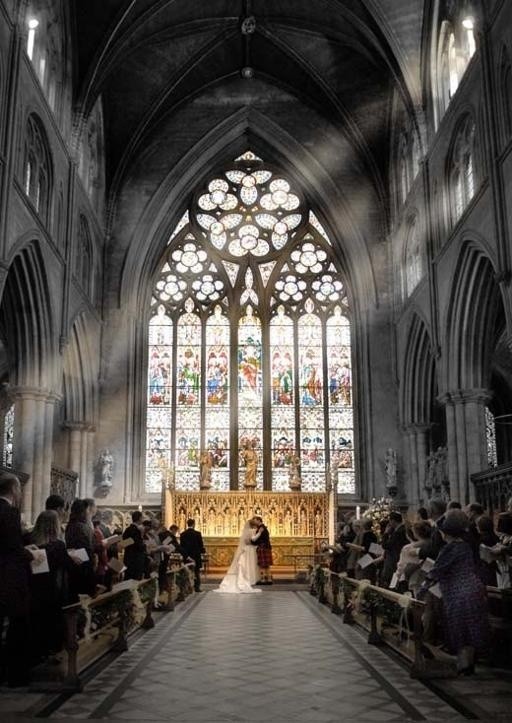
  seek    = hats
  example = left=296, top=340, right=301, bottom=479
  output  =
left=438, top=518, right=471, bottom=541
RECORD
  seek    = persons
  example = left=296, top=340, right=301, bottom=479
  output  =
left=1, top=473, right=206, bottom=685
left=240, top=442, right=259, bottom=483
left=286, top=447, right=301, bottom=481
left=384, top=448, right=400, bottom=486
left=198, top=449, right=213, bottom=486
left=97, top=448, right=114, bottom=482
left=323, top=493, right=511, bottom=679
left=235, top=518, right=268, bottom=586
left=251, top=515, right=275, bottom=585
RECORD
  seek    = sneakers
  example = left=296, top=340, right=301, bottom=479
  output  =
left=446, top=662, right=475, bottom=679
left=256, top=581, right=272, bottom=585
